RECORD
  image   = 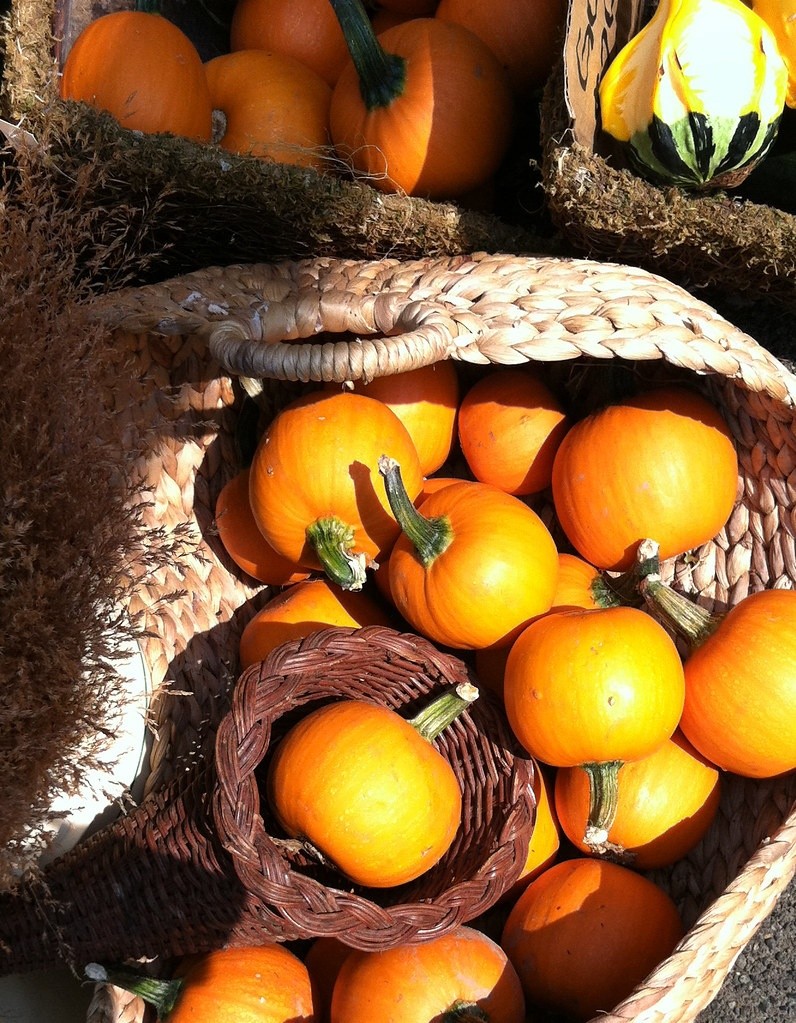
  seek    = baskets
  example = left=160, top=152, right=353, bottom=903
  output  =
left=25, top=256, right=796, bottom=1023
left=0, top=625, right=536, bottom=976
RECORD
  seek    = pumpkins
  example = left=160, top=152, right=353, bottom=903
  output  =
left=89, top=360, right=796, bottom=1023
left=55, top=1, right=558, bottom=199
